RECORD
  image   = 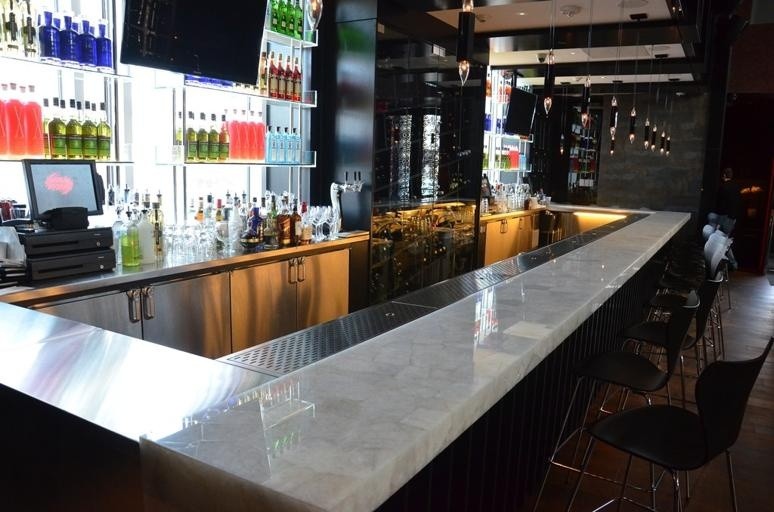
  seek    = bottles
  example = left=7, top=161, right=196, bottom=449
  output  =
left=485, top=112, right=512, bottom=134
left=0, top=83, right=43, bottom=156
left=37, top=11, right=112, bottom=68
left=482, top=145, right=525, bottom=170
left=266, top=126, right=301, bottom=162
left=184, top=74, right=233, bottom=86
left=260, top=52, right=301, bottom=102
left=43, top=97, right=111, bottom=160
left=3, top=11, right=37, bottom=53
left=494, top=182, right=531, bottom=211
left=107, top=185, right=163, bottom=267
left=176, top=111, right=229, bottom=161
left=187, top=191, right=312, bottom=247
left=270, top=0, right=303, bottom=40
left=223, top=107, right=264, bottom=160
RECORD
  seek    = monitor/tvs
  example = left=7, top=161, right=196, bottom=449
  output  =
left=22, top=158, right=103, bottom=221
left=119, top=0, right=267, bottom=86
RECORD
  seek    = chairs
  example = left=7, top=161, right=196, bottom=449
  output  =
left=717, top=216, right=737, bottom=235
left=612, top=232, right=735, bottom=410
left=566, top=336, right=773, bottom=512
left=707, top=212, right=718, bottom=225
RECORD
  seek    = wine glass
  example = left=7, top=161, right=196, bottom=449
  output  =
left=309, top=206, right=331, bottom=239
left=215, top=221, right=240, bottom=254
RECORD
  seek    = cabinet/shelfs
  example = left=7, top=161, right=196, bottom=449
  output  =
left=231, top=234, right=371, bottom=352
left=1, top=0, right=136, bottom=208
left=478, top=214, right=531, bottom=264
left=481, top=65, right=536, bottom=183
left=155, top=0, right=321, bottom=171
left=17, top=258, right=230, bottom=359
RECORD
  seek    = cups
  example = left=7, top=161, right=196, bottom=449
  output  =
left=163, top=220, right=214, bottom=260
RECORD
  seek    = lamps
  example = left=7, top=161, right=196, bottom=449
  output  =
left=543, top=0, right=691, bottom=155
left=532, top=289, right=700, bottom=512
left=309, top=1, right=325, bottom=34
left=459, top=0, right=474, bottom=86
left=580, top=271, right=723, bottom=466
left=646, top=224, right=735, bottom=380
